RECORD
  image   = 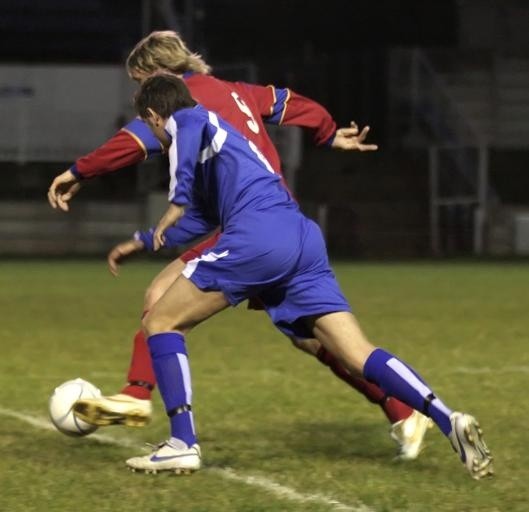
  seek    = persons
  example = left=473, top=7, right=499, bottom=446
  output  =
left=48, top=30, right=432, bottom=462
left=107, top=72, right=496, bottom=481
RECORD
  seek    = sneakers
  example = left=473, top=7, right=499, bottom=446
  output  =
left=388, top=407, right=433, bottom=463
left=125, top=439, right=201, bottom=475
left=450, top=411, right=494, bottom=480
left=71, top=394, right=153, bottom=428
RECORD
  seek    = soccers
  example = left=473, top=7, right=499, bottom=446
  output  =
left=47, top=378, right=104, bottom=437
